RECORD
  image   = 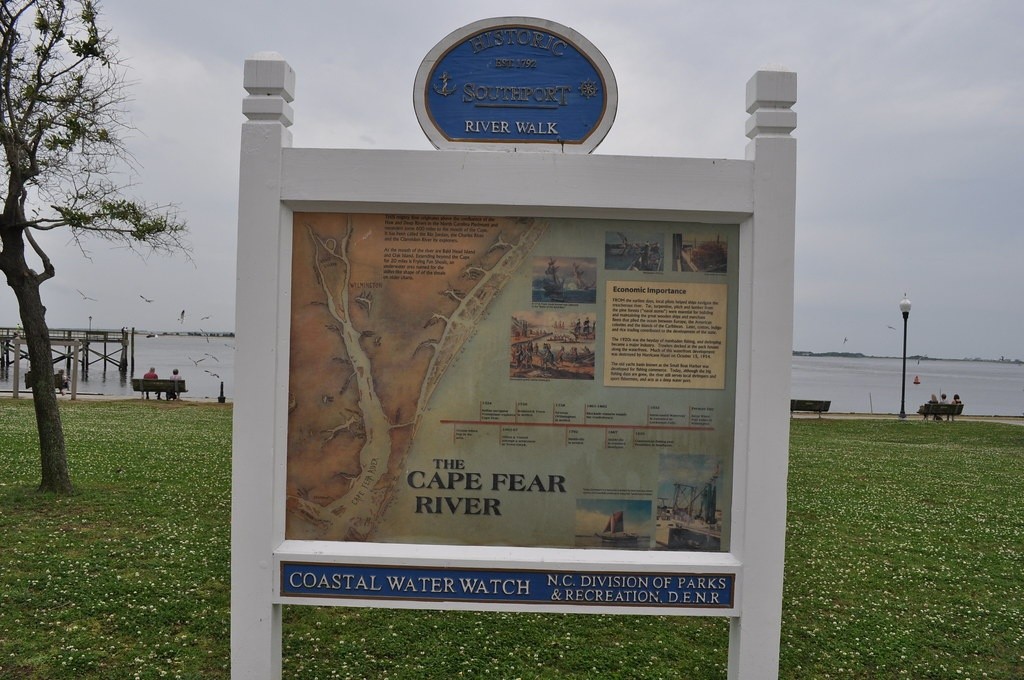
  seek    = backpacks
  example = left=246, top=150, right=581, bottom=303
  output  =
left=936, top=416, right=943, bottom=423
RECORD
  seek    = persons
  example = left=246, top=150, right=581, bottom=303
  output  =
left=168, top=369, right=181, bottom=400
left=144, top=367, right=162, bottom=399
left=922, top=394, right=962, bottom=421
left=121, top=327, right=128, bottom=339
left=58, top=369, right=70, bottom=396
left=16, top=324, right=23, bottom=337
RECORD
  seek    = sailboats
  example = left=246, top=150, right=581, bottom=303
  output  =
left=594, top=511, right=639, bottom=542
left=913, top=375, right=921, bottom=384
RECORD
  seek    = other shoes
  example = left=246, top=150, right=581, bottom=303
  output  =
left=157, top=398, right=162, bottom=400
left=146, top=396, right=149, bottom=399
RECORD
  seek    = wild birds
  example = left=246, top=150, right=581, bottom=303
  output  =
left=841, top=324, right=930, bottom=366
left=75, top=287, right=236, bottom=380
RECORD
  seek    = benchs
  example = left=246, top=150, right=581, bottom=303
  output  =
left=918, top=403, right=965, bottom=421
left=131, top=378, right=189, bottom=400
left=790, top=399, right=832, bottom=418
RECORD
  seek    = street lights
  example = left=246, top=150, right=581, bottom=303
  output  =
left=896, top=292, right=913, bottom=419
left=89, top=316, right=92, bottom=331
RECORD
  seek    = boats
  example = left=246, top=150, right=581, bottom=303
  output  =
left=146, top=334, right=158, bottom=338
